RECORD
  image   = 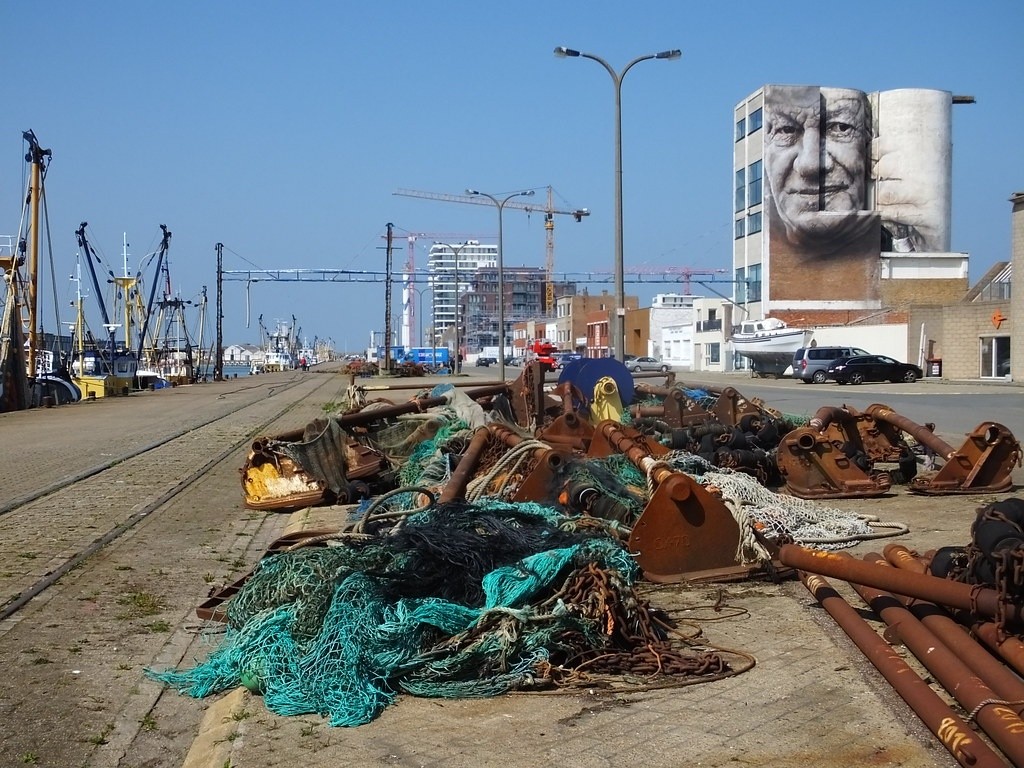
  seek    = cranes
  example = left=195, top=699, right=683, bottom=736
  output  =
left=390, top=184, right=592, bottom=319
left=380, top=233, right=500, bottom=347
left=592, top=261, right=729, bottom=297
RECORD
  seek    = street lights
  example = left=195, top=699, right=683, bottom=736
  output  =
left=432, top=240, right=480, bottom=374
left=406, top=286, right=440, bottom=347
left=414, top=267, right=449, bottom=368
left=463, top=189, right=537, bottom=381
left=392, top=313, right=409, bottom=346
left=551, top=44, right=683, bottom=366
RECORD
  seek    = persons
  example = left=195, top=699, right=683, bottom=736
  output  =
left=300, top=355, right=311, bottom=371
left=450, top=353, right=463, bottom=373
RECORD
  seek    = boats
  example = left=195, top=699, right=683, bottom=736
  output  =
left=0, top=127, right=217, bottom=411
left=246, top=313, right=336, bottom=376
left=725, top=317, right=815, bottom=380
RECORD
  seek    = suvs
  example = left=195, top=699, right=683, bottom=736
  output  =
left=792, top=345, right=887, bottom=384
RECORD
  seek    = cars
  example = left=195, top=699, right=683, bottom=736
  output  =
left=488, top=337, right=583, bottom=372
left=826, top=354, right=925, bottom=385
left=608, top=354, right=638, bottom=362
left=475, top=358, right=490, bottom=368
left=625, top=355, right=672, bottom=373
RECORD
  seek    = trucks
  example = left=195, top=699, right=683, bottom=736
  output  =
left=396, top=347, right=450, bottom=375
